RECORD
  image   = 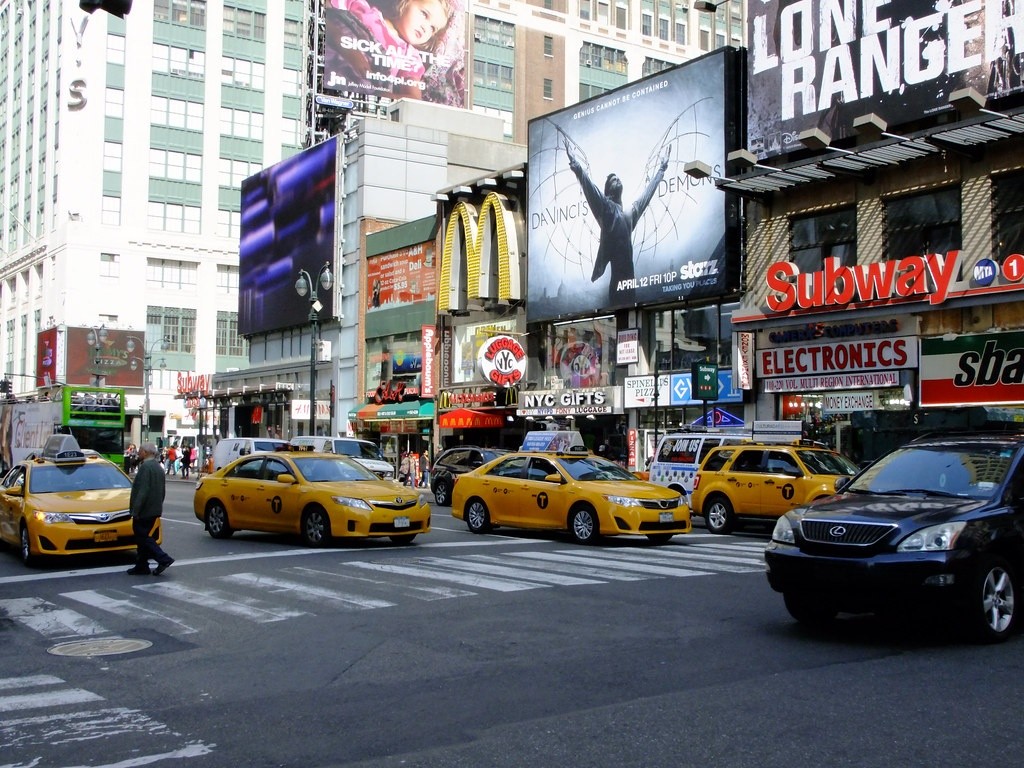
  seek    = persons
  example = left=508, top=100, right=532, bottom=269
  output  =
left=600, top=444, right=618, bottom=461
left=124, top=443, right=213, bottom=480
left=351, top=443, right=366, bottom=456
left=5, top=390, right=118, bottom=413
left=399, top=445, right=444, bottom=490
left=127, top=442, right=175, bottom=576
left=84, top=466, right=113, bottom=488
left=316, top=460, right=341, bottom=480
left=582, top=459, right=603, bottom=481
left=549, top=436, right=570, bottom=452
left=323, top=0, right=455, bottom=100
left=563, top=138, right=671, bottom=306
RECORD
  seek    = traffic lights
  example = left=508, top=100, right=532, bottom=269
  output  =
left=0, top=380, right=12, bottom=393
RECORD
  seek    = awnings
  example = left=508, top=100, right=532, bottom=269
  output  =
left=348, top=401, right=434, bottom=421
left=439, top=408, right=503, bottom=428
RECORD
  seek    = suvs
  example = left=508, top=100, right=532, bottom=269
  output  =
left=691, top=439, right=862, bottom=533
left=764, top=421, right=1024, bottom=644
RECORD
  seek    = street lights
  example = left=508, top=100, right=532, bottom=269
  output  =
left=295, top=260, right=334, bottom=435
left=87, top=323, right=109, bottom=395
left=127, top=336, right=170, bottom=442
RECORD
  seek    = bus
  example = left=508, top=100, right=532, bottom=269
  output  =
left=0, top=384, right=127, bottom=483
left=165, top=428, right=220, bottom=467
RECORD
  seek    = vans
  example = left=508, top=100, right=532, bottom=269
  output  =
left=290, top=434, right=395, bottom=480
left=212, top=436, right=313, bottom=477
left=648, top=432, right=830, bottom=514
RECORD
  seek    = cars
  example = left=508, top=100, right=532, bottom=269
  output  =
left=431, top=445, right=515, bottom=505
left=194, top=452, right=432, bottom=548
left=450, top=430, right=689, bottom=544
left=0, top=434, right=161, bottom=566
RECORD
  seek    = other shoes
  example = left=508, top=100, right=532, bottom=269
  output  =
left=152, top=559, right=175, bottom=575
left=166, top=473, right=169, bottom=475
left=173, top=474, right=175, bottom=475
left=127, top=566, right=151, bottom=575
